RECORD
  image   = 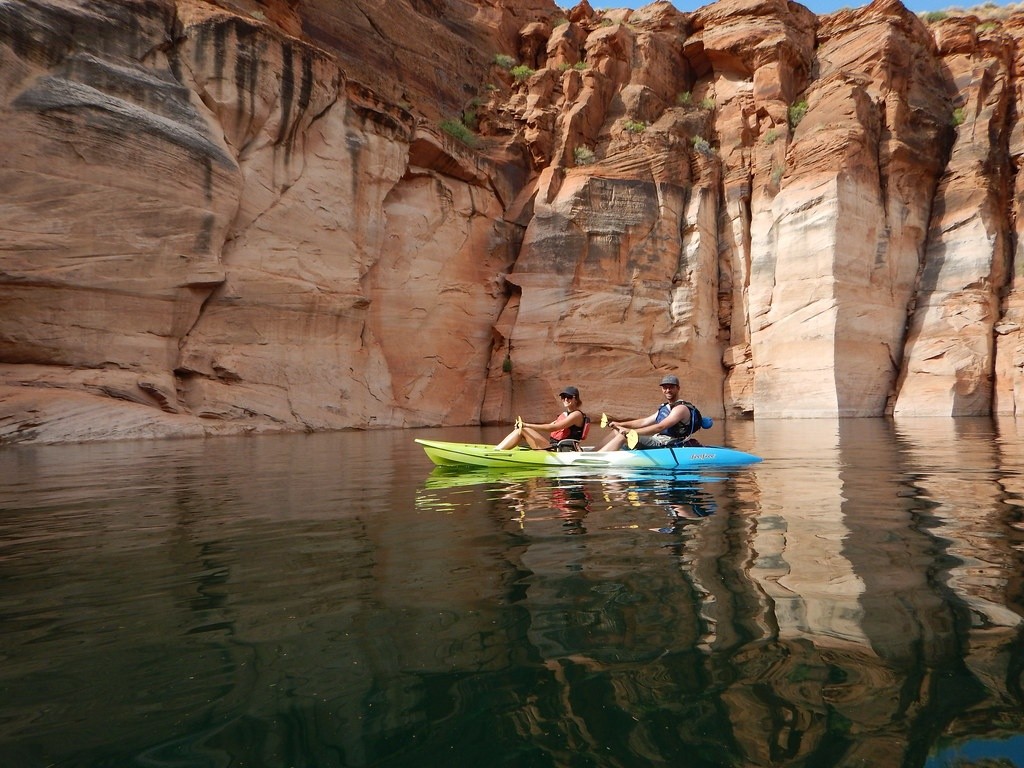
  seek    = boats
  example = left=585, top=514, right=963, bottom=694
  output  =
left=414, top=438, right=763, bottom=469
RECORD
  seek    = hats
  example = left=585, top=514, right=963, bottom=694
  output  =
left=659, top=375, right=679, bottom=386
left=559, top=386, right=579, bottom=399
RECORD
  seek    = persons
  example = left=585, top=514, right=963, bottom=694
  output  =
left=589, top=374, right=692, bottom=452
left=495, top=385, right=585, bottom=452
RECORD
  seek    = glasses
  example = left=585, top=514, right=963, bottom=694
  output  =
left=561, top=394, right=576, bottom=400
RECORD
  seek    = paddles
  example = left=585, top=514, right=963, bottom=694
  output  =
left=515, top=415, right=523, bottom=437
left=599, top=412, right=639, bottom=450
